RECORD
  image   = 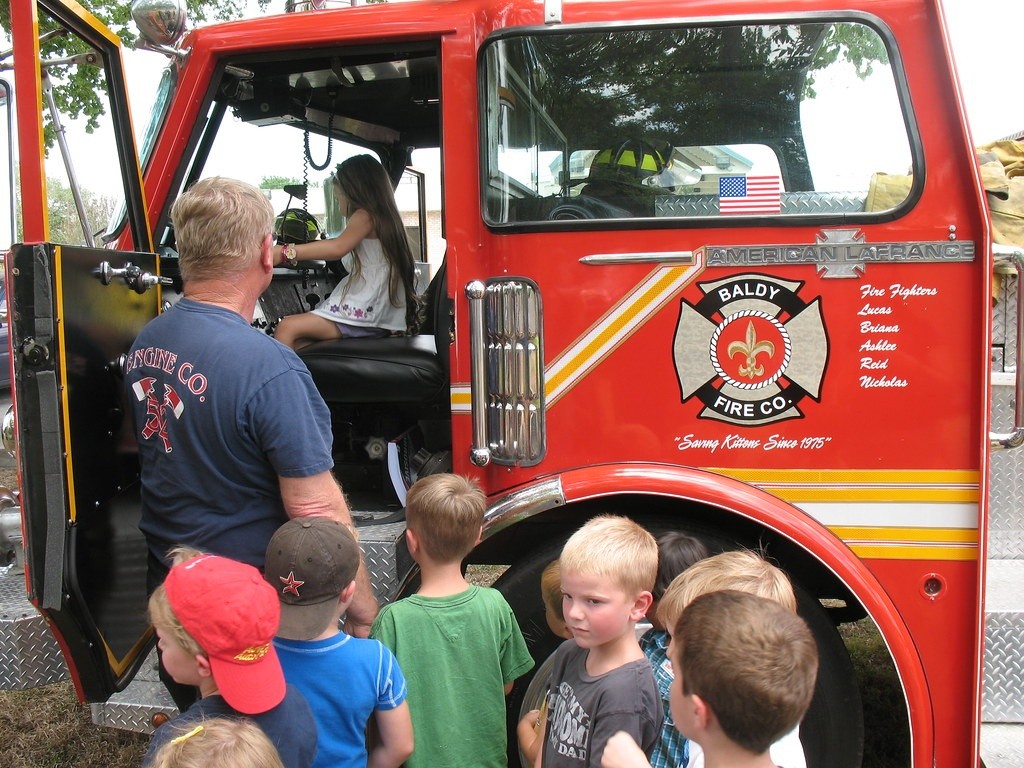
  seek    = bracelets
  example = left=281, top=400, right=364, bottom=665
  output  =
left=280, top=243, right=291, bottom=265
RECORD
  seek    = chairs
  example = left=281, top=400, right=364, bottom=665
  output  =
left=296, top=253, right=448, bottom=402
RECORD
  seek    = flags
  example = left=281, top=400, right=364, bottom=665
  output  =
left=719, top=176, right=781, bottom=216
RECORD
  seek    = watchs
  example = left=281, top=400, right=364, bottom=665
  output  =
left=286, top=243, right=299, bottom=266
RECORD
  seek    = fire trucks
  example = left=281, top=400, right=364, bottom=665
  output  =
left=3, top=1, right=1024, bottom=767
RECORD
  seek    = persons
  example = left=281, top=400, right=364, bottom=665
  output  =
left=271, top=153, right=424, bottom=354
left=138, top=545, right=320, bottom=768
left=657, top=546, right=809, bottom=768
left=665, top=588, right=818, bottom=767
left=520, top=558, right=575, bottom=765
left=534, top=513, right=665, bottom=768
left=367, top=473, right=536, bottom=768
left=124, top=177, right=381, bottom=715
left=637, top=530, right=707, bottom=768
left=140, top=716, right=285, bottom=768
left=263, top=516, right=416, bottom=768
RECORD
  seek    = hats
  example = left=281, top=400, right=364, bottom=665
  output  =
left=265, top=515, right=361, bottom=640
left=164, top=556, right=286, bottom=714
